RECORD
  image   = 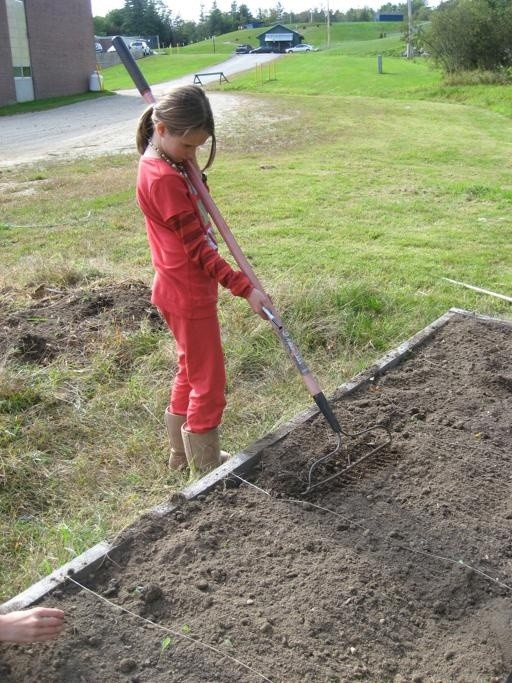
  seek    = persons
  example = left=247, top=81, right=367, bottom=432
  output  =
left=0, top=604, right=65, bottom=646
left=134, top=81, right=276, bottom=491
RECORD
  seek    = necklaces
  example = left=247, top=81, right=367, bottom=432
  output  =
left=147, top=137, right=189, bottom=178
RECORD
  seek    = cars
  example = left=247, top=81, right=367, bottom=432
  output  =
left=284, top=43, right=313, bottom=52
left=248, top=45, right=280, bottom=54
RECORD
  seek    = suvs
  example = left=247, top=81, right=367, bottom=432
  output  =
left=95, top=39, right=151, bottom=56
left=236, top=43, right=252, bottom=54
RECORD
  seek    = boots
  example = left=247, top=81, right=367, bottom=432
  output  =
left=181, top=422, right=221, bottom=482
left=165, top=406, right=231, bottom=471
